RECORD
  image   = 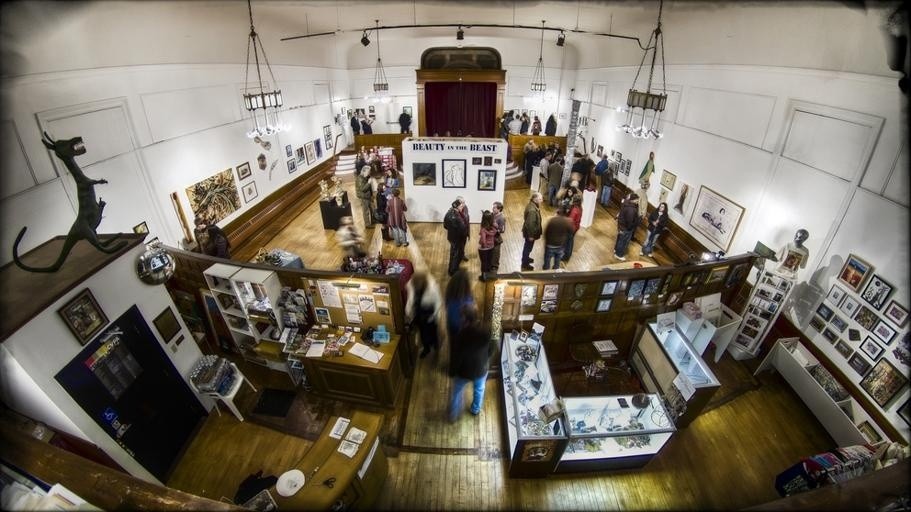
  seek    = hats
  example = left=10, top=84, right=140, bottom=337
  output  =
left=451, top=199, right=462, bottom=208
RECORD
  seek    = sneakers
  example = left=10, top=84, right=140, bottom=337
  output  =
left=381, top=228, right=409, bottom=247
left=613, top=250, right=653, bottom=261
left=521, top=257, right=534, bottom=271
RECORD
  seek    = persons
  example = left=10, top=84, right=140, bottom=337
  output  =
left=444, top=195, right=506, bottom=282
left=447, top=302, right=494, bottom=421
left=400, top=109, right=412, bottom=134
left=351, top=112, right=374, bottom=135
left=567, top=152, right=614, bottom=206
left=614, top=181, right=668, bottom=263
left=522, top=186, right=583, bottom=272
left=522, top=139, right=565, bottom=207
left=499, top=110, right=557, bottom=136
left=355, top=152, right=409, bottom=247
left=777, top=230, right=809, bottom=278
left=205, top=226, right=231, bottom=259
left=404, top=272, right=441, bottom=361
left=446, top=271, right=475, bottom=338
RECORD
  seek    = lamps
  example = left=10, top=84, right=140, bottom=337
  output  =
left=243, top=1, right=284, bottom=139
left=280, top=0, right=655, bottom=50
left=524, top=21, right=555, bottom=104
left=363, top=20, right=393, bottom=104
left=618, top=1, right=667, bottom=140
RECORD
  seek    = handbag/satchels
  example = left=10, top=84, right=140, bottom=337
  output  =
left=371, top=209, right=389, bottom=225
